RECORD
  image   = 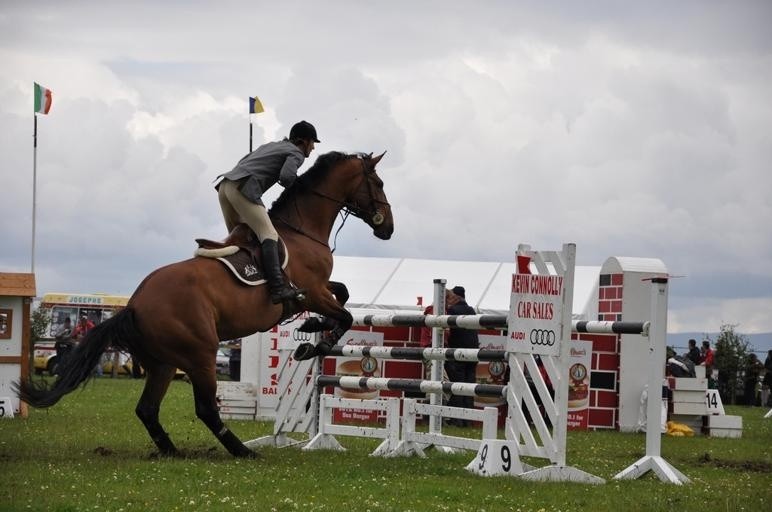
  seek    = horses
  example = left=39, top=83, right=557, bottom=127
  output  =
left=7, top=149, right=395, bottom=460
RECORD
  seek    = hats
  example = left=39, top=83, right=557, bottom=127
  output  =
left=451, top=285, right=467, bottom=300
left=289, top=119, right=322, bottom=145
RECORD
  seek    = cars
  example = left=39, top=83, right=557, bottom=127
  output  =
left=216, top=347, right=231, bottom=374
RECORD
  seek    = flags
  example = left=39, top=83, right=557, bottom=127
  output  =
left=34, top=83, right=53, bottom=115
left=248, top=96, right=265, bottom=114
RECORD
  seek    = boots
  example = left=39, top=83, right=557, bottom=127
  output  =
left=261, top=237, right=305, bottom=305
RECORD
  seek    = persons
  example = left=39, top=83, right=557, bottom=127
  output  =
left=213, top=119, right=322, bottom=304
left=686, top=338, right=701, bottom=367
left=699, top=340, right=713, bottom=383
left=762, top=349, right=772, bottom=406
left=417, top=288, right=453, bottom=427
left=437, top=285, right=481, bottom=430
left=70, top=316, right=104, bottom=376
left=54, top=316, right=77, bottom=358
left=741, top=353, right=764, bottom=405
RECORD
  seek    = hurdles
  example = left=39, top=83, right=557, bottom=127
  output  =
left=242, top=243, right=693, bottom=485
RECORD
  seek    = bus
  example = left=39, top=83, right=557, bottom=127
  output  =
left=34, top=295, right=190, bottom=375
